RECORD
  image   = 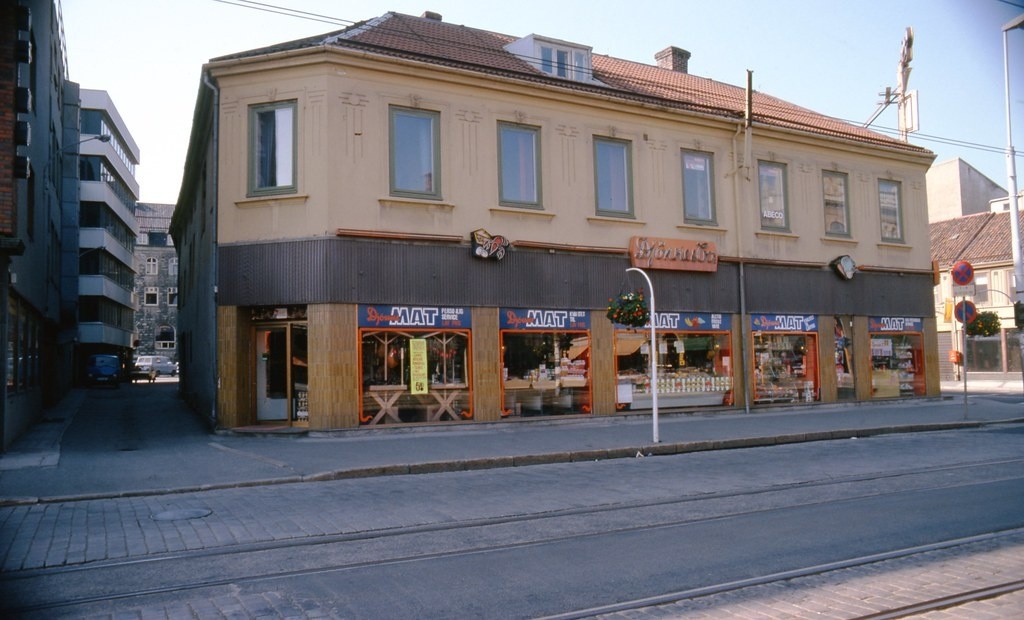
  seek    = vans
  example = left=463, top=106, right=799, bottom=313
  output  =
left=135, top=356, right=177, bottom=378
left=85, top=354, right=122, bottom=389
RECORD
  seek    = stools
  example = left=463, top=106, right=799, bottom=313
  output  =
left=505, top=376, right=588, bottom=416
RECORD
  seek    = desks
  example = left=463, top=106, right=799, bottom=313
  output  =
left=426, top=383, right=469, bottom=421
left=366, top=384, right=408, bottom=425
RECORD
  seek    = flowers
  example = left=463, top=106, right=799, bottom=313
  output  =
left=606, top=287, right=650, bottom=326
left=961, top=310, right=1003, bottom=338
